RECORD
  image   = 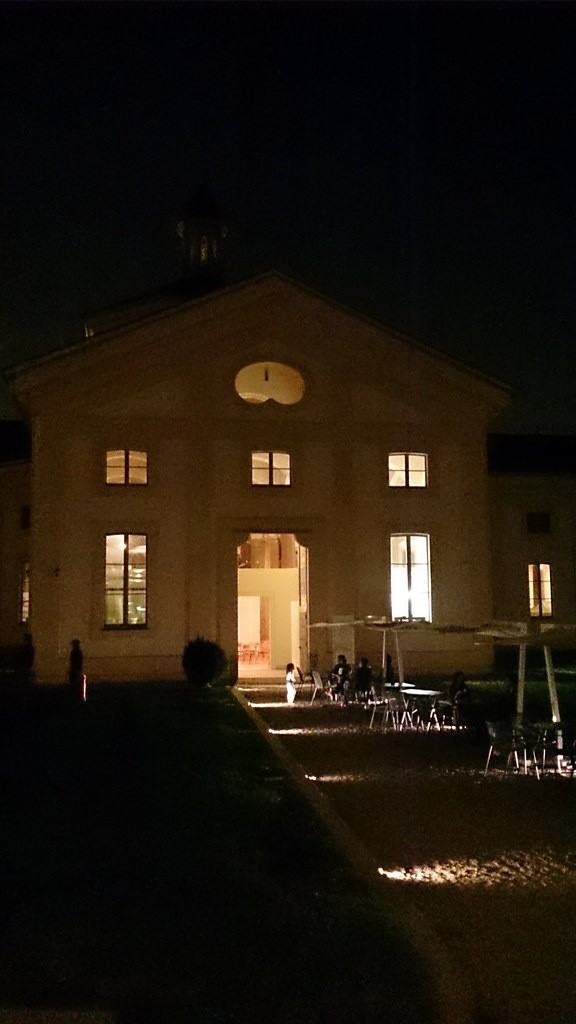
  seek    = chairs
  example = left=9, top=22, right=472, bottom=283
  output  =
left=238, top=641, right=270, bottom=664
left=296, top=667, right=576, bottom=782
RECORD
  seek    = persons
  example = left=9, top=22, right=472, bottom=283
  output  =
left=333, top=654, right=394, bottom=702
left=285, top=663, right=298, bottom=708
left=450, top=671, right=490, bottom=741
left=17, top=633, right=35, bottom=686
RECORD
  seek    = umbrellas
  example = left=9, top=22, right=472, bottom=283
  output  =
left=469, top=618, right=576, bottom=762
left=306, top=617, right=488, bottom=695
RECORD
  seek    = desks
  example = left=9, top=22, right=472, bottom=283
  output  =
left=399, top=688, right=443, bottom=732
left=382, top=682, right=416, bottom=731
